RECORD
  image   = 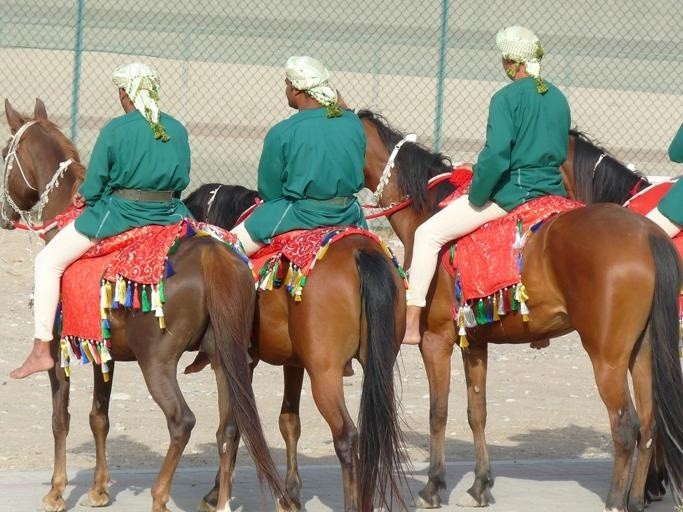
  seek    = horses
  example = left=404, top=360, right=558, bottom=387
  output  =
left=0, top=98, right=299, bottom=512
left=344, top=108, right=683, bottom=512
left=181, top=184, right=420, bottom=512
left=559, top=120, right=682, bottom=326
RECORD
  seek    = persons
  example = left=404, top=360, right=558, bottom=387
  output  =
left=396, top=26, right=572, bottom=346
left=185, top=57, right=363, bottom=375
left=639, top=124, right=683, bottom=239
left=9, top=64, right=190, bottom=381
